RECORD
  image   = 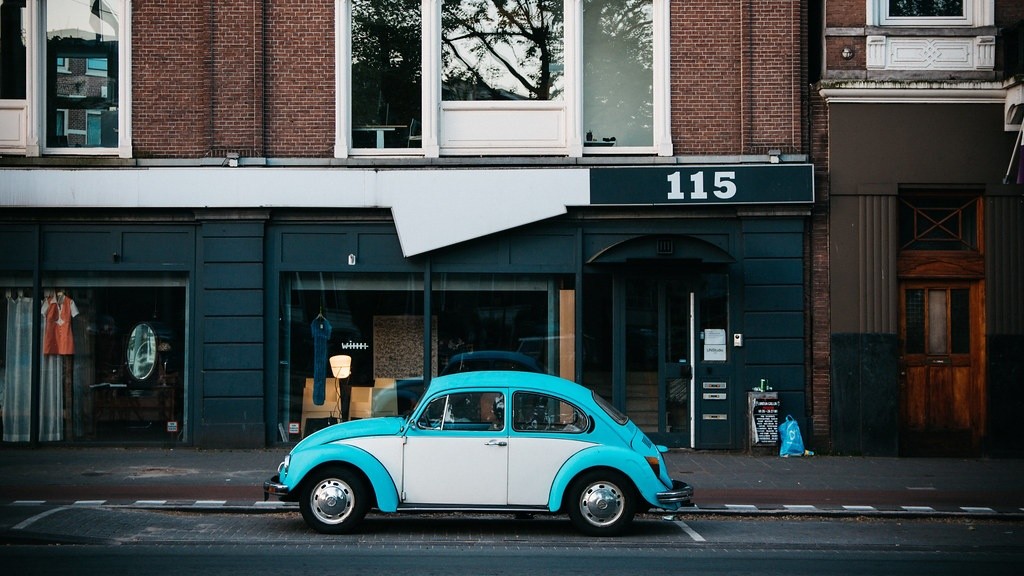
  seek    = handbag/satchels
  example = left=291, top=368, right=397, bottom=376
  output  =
left=778, top=414, right=805, bottom=457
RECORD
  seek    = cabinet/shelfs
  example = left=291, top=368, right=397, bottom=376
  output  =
left=95, top=364, right=175, bottom=439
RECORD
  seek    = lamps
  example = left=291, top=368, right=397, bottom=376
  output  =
left=330, top=354, right=352, bottom=379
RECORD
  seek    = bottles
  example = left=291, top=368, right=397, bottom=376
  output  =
left=752, top=378, right=773, bottom=392
left=804, top=450, right=814, bottom=456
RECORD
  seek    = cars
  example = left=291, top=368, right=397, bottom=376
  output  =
left=262, top=369, right=693, bottom=540
left=370, top=349, right=542, bottom=437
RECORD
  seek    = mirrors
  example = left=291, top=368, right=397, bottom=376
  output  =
left=124, top=322, right=158, bottom=382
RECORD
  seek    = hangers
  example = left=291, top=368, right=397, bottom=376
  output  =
left=46, top=289, right=73, bottom=308
left=311, top=306, right=332, bottom=328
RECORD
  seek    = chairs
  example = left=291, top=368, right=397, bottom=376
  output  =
left=348, top=378, right=398, bottom=421
left=300, top=378, right=343, bottom=438
